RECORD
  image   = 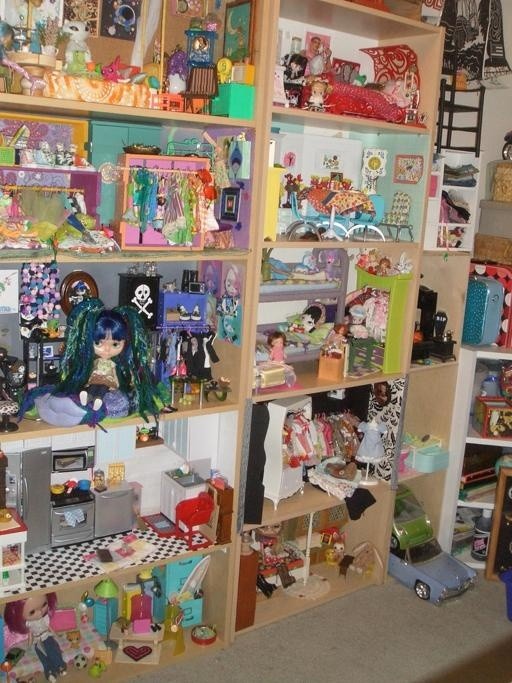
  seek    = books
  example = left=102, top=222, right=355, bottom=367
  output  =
left=459, top=476, right=498, bottom=500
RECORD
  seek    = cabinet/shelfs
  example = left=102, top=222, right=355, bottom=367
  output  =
left=0, top=0, right=512, bottom=683
